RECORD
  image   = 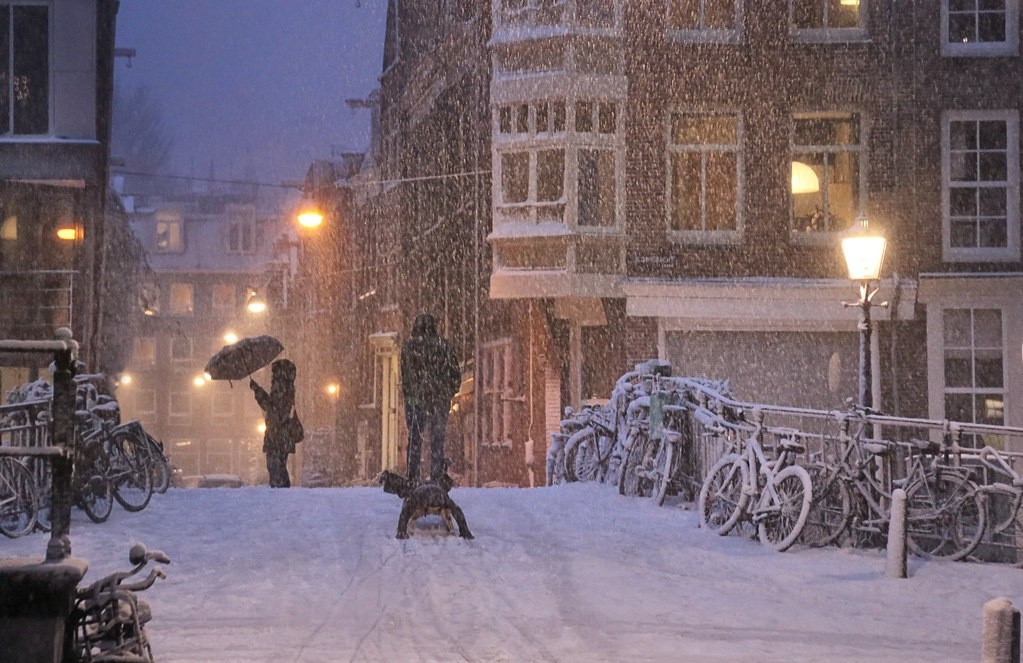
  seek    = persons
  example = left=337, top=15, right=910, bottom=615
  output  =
left=249, top=357, right=296, bottom=490
left=401, top=314, right=460, bottom=490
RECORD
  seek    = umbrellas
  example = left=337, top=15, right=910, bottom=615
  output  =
left=203, top=335, right=286, bottom=390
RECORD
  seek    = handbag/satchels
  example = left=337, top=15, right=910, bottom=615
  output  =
left=288, top=400, right=304, bottom=443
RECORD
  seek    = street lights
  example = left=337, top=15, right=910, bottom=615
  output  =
left=838, top=209, right=894, bottom=549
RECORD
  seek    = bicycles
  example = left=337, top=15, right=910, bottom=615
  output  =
left=546, top=357, right=1023, bottom=570
left=64, top=543, right=171, bottom=663
left=0, top=356, right=170, bottom=539
